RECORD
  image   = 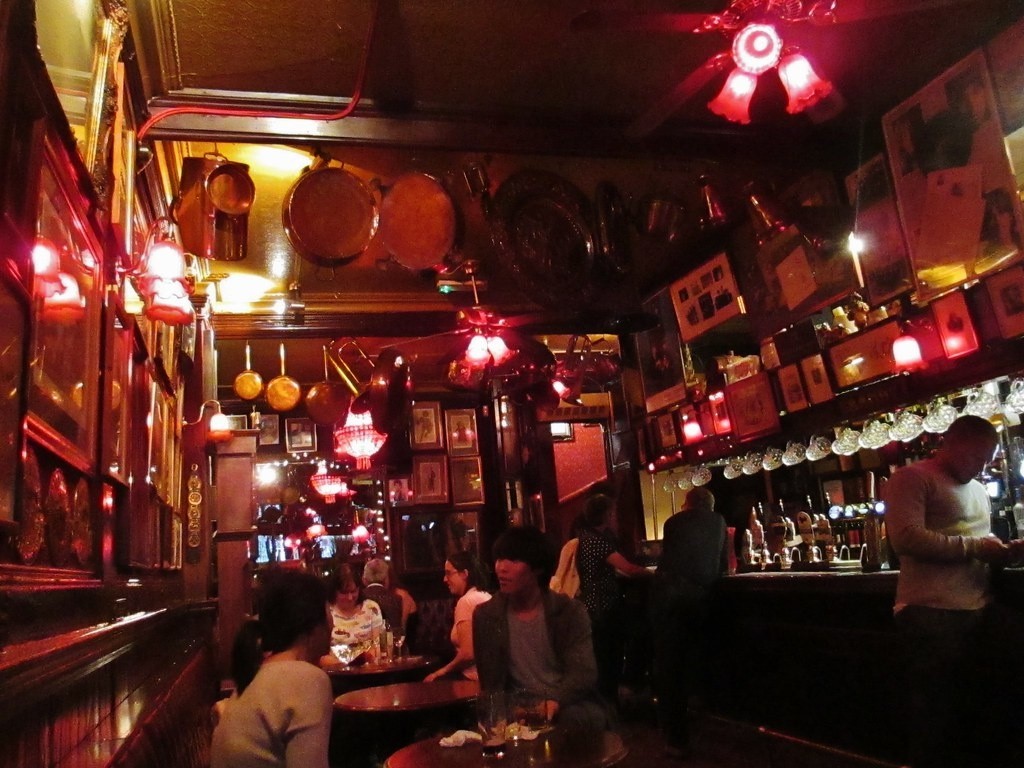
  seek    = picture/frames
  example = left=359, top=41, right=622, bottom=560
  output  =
left=395, top=511, right=479, bottom=574
left=222, top=413, right=247, bottom=432
left=285, top=416, right=318, bottom=453
left=451, top=457, right=485, bottom=506
left=383, top=475, right=412, bottom=508
left=0, top=117, right=204, bottom=592
left=408, top=398, right=443, bottom=451
left=258, top=411, right=281, bottom=445
left=636, top=19, right=1024, bottom=463
left=549, top=420, right=577, bottom=443
left=413, top=454, right=450, bottom=504
left=445, top=408, right=479, bottom=456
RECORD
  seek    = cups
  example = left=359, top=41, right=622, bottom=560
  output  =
left=664, top=377, right=1023, bottom=492
left=475, top=686, right=548, bottom=758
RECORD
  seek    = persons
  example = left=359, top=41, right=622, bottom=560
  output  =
left=392, top=480, right=404, bottom=500
left=421, top=551, right=492, bottom=723
left=548, top=493, right=647, bottom=720
left=427, top=467, right=436, bottom=495
left=455, top=422, right=470, bottom=445
left=644, top=302, right=682, bottom=395
left=419, top=410, right=432, bottom=443
left=650, top=487, right=726, bottom=762
left=226, top=547, right=416, bottom=698
left=472, top=527, right=603, bottom=760
left=211, top=571, right=335, bottom=767
left=886, top=416, right=1024, bottom=768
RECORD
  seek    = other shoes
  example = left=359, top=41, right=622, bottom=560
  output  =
left=665, top=745, right=692, bottom=759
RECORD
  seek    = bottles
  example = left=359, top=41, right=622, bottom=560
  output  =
left=832, top=521, right=865, bottom=548
left=756, top=492, right=833, bottom=537
left=380, top=618, right=394, bottom=663
left=1013, top=487, right=1024, bottom=539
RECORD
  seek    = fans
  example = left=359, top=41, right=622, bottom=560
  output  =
left=646, top=0, right=920, bottom=111
left=380, top=253, right=540, bottom=354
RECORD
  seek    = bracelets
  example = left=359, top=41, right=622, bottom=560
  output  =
left=431, top=672, right=440, bottom=681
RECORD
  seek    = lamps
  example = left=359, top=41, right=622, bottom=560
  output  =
left=466, top=334, right=523, bottom=369
left=131, top=272, right=196, bottom=327
left=332, top=387, right=388, bottom=471
left=891, top=313, right=941, bottom=371
left=183, top=398, right=230, bottom=435
left=695, top=24, right=863, bottom=125
left=520, top=328, right=622, bottom=404
left=114, top=215, right=194, bottom=281
left=311, top=458, right=342, bottom=504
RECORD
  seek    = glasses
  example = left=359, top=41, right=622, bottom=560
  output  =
left=445, top=569, right=458, bottom=579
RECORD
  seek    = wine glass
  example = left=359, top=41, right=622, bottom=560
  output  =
left=394, top=636, right=407, bottom=664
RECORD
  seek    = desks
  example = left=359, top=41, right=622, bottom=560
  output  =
left=331, top=678, right=481, bottom=712
left=323, top=655, right=440, bottom=675
left=381, top=719, right=630, bottom=768
